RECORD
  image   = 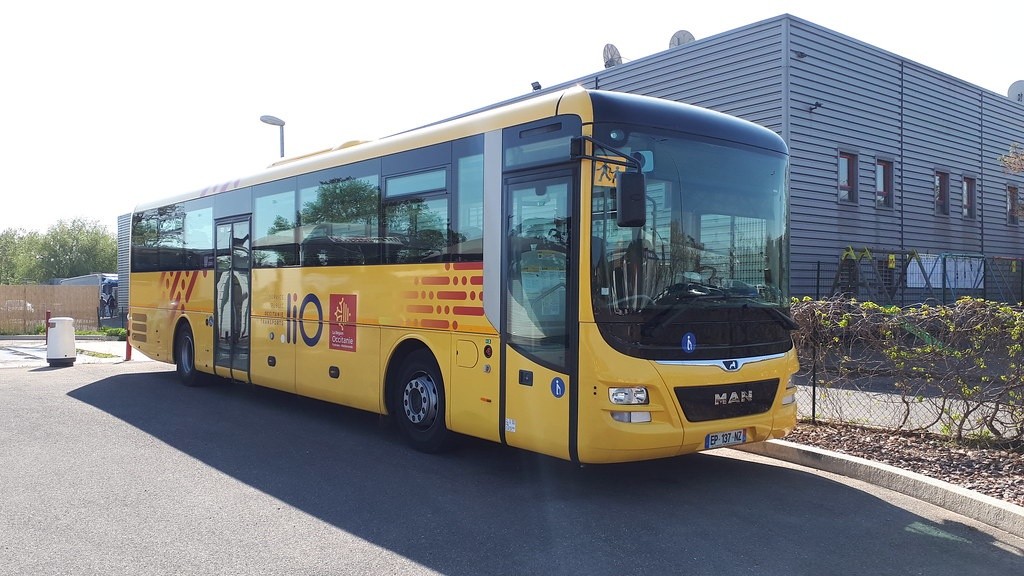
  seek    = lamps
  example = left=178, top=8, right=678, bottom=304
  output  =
left=810, top=102, right=823, bottom=111
left=532, top=82, right=542, bottom=92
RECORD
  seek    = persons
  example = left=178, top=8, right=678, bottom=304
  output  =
left=100, top=295, right=107, bottom=319
left=107, top=294, right=115, bottom=318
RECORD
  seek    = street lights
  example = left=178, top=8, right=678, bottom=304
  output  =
left=260, top=114, right=287, bottom=157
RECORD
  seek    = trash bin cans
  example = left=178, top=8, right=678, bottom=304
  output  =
left=45, top=317, right=78, bottom=367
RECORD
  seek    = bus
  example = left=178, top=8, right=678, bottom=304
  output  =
left=128, top=86, right=802, bottom=464
